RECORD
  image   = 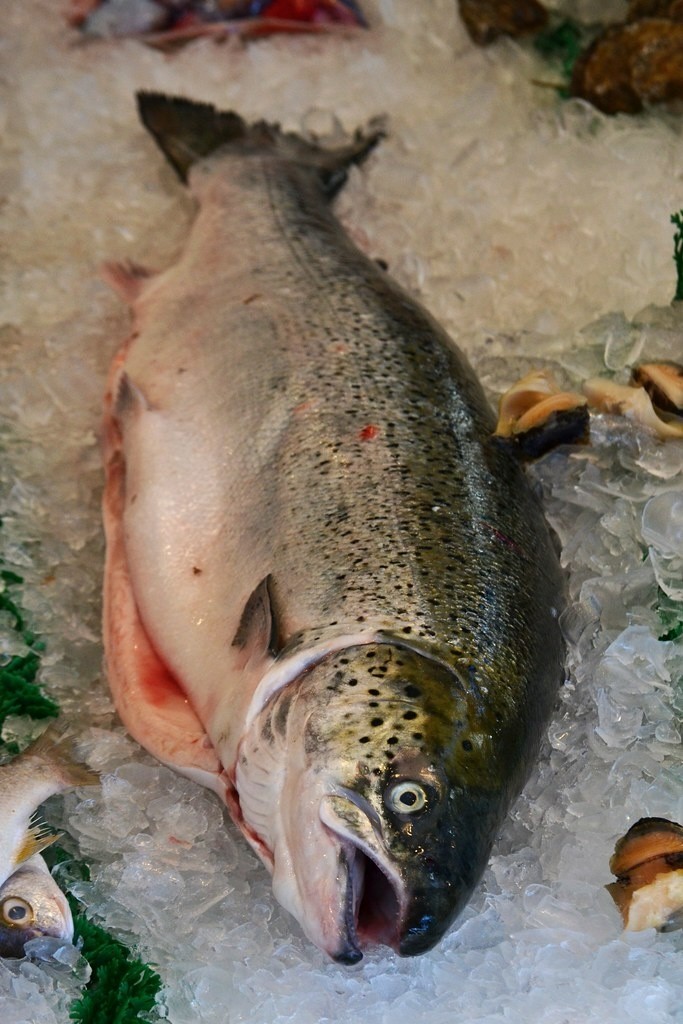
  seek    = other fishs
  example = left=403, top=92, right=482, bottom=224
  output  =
left=95, top=85, right=565, bottom=964
left=0, top=720, right=105, bottom=962
